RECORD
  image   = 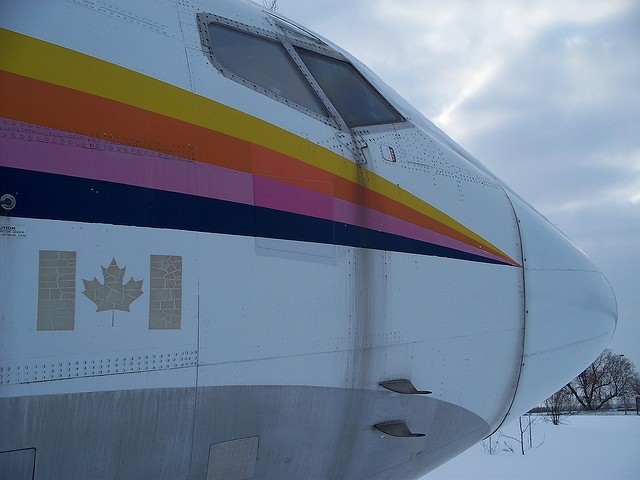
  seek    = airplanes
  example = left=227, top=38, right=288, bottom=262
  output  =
left=0, top=0, right=619, bottom=479
